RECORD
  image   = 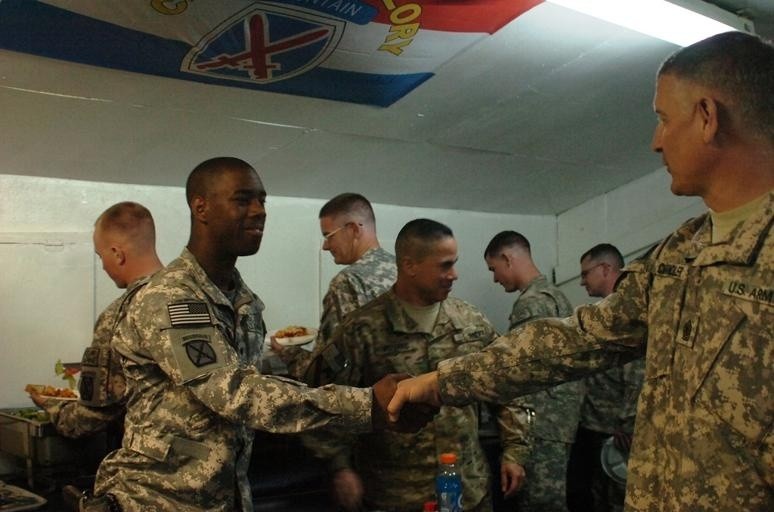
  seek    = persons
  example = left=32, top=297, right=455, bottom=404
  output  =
left=579, top=244, right=646, bottom=511
left=270, top=194, right=400, bottom=382
left=485, top=231, right=588, bottom=511
left=388, top=32, right=774, bottom=511
left=302, top=218, right=536, bottom=511
left=28, top=202, right=165, bottom=452
left=94, top=157, right=439, bottom=512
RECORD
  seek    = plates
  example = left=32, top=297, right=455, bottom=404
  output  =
left=0, top=484, right=47, bottom=512
left=25, top=389, right=81, bottom=401
left=263, top=328, right=318, bottom=346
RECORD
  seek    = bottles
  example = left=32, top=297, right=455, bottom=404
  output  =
left=435, top=453, right=464, bottom=511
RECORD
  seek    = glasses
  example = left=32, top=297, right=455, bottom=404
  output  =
left=579, top=262, right=611, bottom=280
left=322, top=221, right=363, bottom=242
